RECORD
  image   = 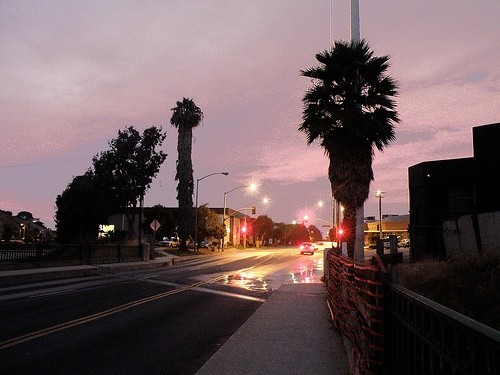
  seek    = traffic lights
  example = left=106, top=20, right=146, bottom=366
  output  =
left=377, top=225, right=379, bottom=230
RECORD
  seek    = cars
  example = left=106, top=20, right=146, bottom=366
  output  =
left=200, top=241, right=212, bottom=248
left=384, top=238, right=409, bottom=249
left=159, top=238, right=179, bottom=249
left=300, top=242, right=318, bottom=255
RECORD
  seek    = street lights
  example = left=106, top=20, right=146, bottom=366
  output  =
left=193, top=171, right=229, bottom=253
left=244, top=197, right=269, bottom=248
left=374, top=191, right=387, bottom=238
left=222, top=184, right=256, bottom=252
left=285, top=220, right=297, bottom=246
left=318, top=202, right=335, bottom=247
